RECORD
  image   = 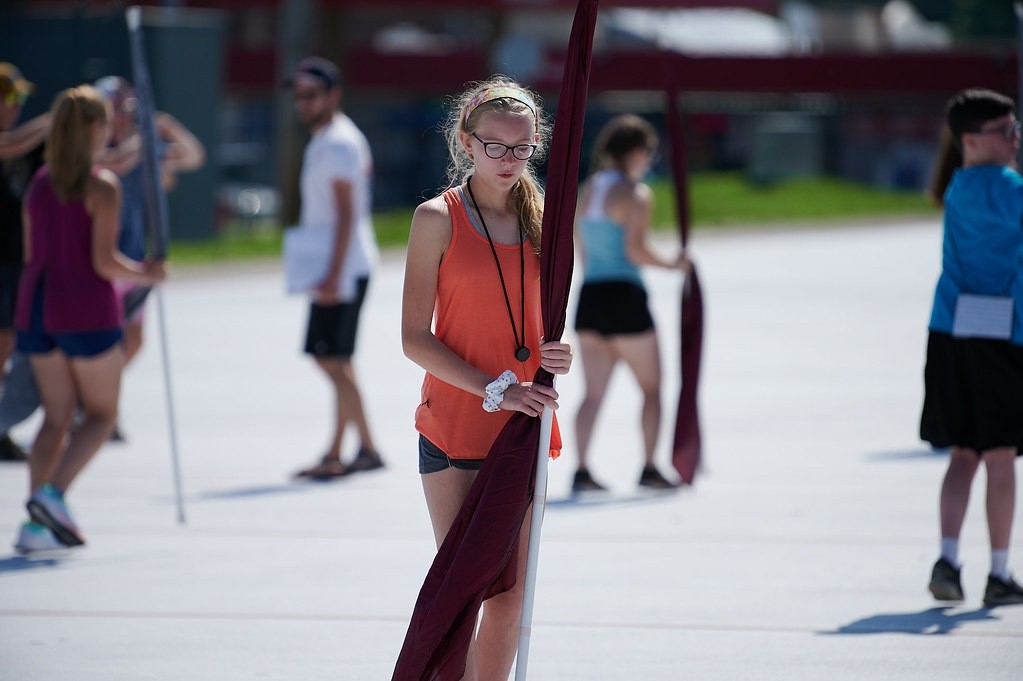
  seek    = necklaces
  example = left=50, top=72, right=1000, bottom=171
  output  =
left=466, top=173, right=531, bottom=363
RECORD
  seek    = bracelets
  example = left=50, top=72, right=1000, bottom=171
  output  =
left=481, top=370, right=518, bottom=412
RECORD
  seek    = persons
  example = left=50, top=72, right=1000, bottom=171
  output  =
left=919, top=92, right=1022, bottom=609
left=13, top=88, right=168, bottom=550
left=0, top=63, right=201, bottom=464
left=401, top=82, right=574, bottom=680
left=280, top=59, right=384, bottom=481
left=568, top=117, right=692, bottom=493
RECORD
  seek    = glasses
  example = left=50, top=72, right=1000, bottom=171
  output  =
left=468, top=131, right=537, bottom=161
left=982, top=121, right=1023, bottom=142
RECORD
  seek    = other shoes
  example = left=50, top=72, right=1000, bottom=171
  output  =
left=28, top=485, right=84, bottom=547
left=299, top=457, right=343, bottom=480
left=930, top=558, right=963, bottom=600
left=345, top=451, right=383, bottom=475
left=16, top=523, right=71, bottom=554
left=571, top=469, right=601, bottom=491
left=638, top=466, right=674, bottom=488
left=0, top=431, right=29, bottom=461
left=983, top=576, right=1023, bottom=605
